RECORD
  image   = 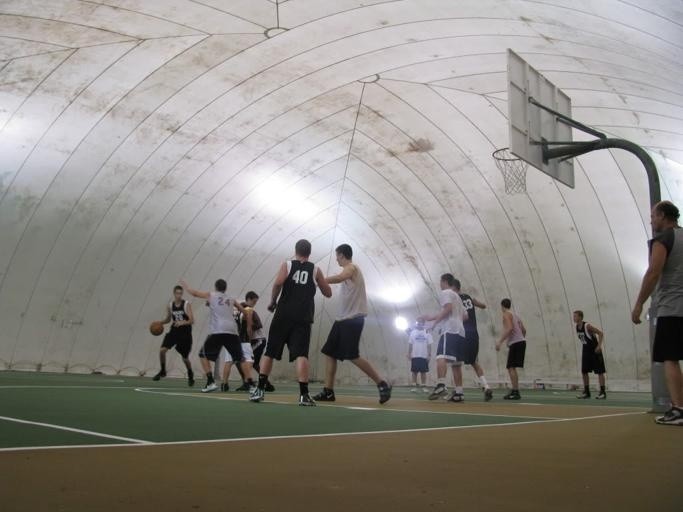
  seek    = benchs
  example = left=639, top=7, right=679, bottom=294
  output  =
left=477, top=379, right=581, bottom=389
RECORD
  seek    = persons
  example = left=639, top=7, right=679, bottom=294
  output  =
left=495, top=297, right=527, bottom=400
left=443, top=279, right=492, bottom=402
left=248, top=239, right=332, bottom=406
left=311, top=244, right=393, bottom=404
left=632, top=201, right=683, bottom=425
left=414, top=273, right=469, bottom=402
left=573, top=311, right=606, bottom=400
left=152, top=277, right=274, bottom=392
left=406, top=318, right=434, bottom=393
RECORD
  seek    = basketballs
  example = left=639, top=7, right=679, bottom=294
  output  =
left=150, top=321, right=163, bottom=335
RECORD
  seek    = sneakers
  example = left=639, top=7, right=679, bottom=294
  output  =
left=406, top=385, right=526, bottom=402
left=150, top=372, right=196, bottom=389
left=575, top=389, right=610, bottom=400
left=658, top=405, right=683, bottom=425
left=301, top=388, right=335, bottom=405
left=375, top=380, right=390, bottom=401
left=200, top=381, right=276, bottom=402
